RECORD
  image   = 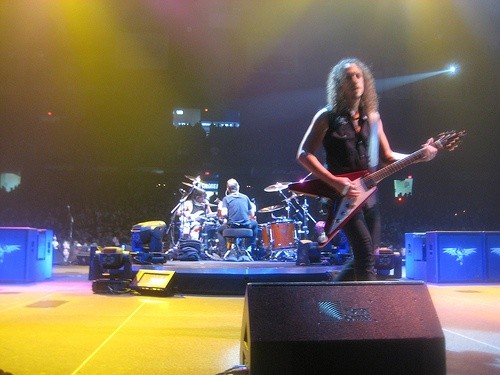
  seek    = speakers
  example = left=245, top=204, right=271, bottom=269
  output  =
left=405, top=231, right=500, bottom=284
left=0, top=227, right=53, bottom=283
left=239, top=280, right=447, bottom=375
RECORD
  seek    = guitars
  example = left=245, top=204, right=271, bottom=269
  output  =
left=288, top=129, right=466, bottom=249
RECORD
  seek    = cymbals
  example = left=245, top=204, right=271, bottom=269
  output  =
left=257, top=204, right=286, bottom=212
left=184, top=174, right=209, bottom=187
left=263, top=182, right=293, bottom=192
left=181, top=181, right=206, bottom=192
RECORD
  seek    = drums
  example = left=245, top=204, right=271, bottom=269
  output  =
left=262, top=218, right=295, bottom=249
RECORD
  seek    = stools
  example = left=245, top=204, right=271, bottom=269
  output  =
left=223, top=229, right=253, bottom=261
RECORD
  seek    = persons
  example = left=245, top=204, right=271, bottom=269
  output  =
left=218, top=178, right=258, bottom=262
left=314, top=221, right=349, bottom=266
left=297, top=59, right=438, bottom=282
left=0, top=186, right=500, bottom=264
left=175, top=187, right=212, bottom=241
